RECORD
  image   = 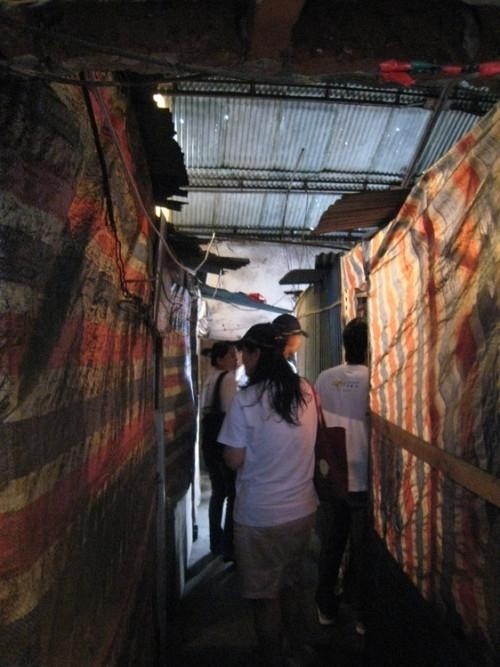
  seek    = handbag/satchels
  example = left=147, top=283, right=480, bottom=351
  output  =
left=201, top=404, right=225, bottom=469
left=314, top=427, right=348, bottom=501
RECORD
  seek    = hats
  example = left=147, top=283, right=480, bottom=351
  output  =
left=234, top=324, right=281, bottom=347
left=273, top=314, right=309, bottom=338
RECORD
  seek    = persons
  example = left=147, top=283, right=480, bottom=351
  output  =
left=311, top=315, right=373, bottom=635
left=213, top=318, right=327, bottom=667
left=199, top=312, right=310, bottom=600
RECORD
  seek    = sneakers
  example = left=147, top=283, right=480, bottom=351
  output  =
left=318, top=607, right=334, bottom=624
left=354, top=619, right=366, bottom=634
